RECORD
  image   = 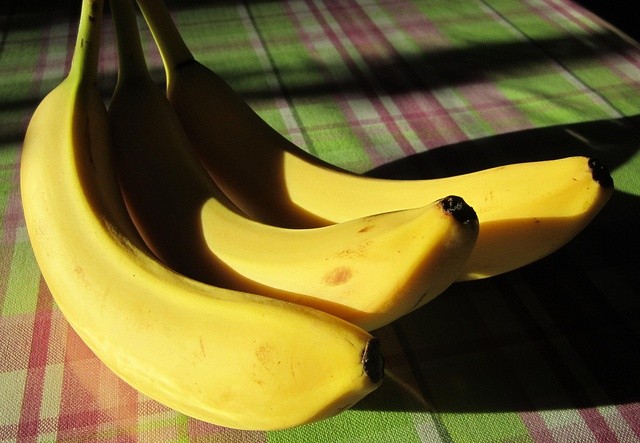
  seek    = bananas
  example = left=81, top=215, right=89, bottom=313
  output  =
left=20, top=0, right=616, bottom=434
